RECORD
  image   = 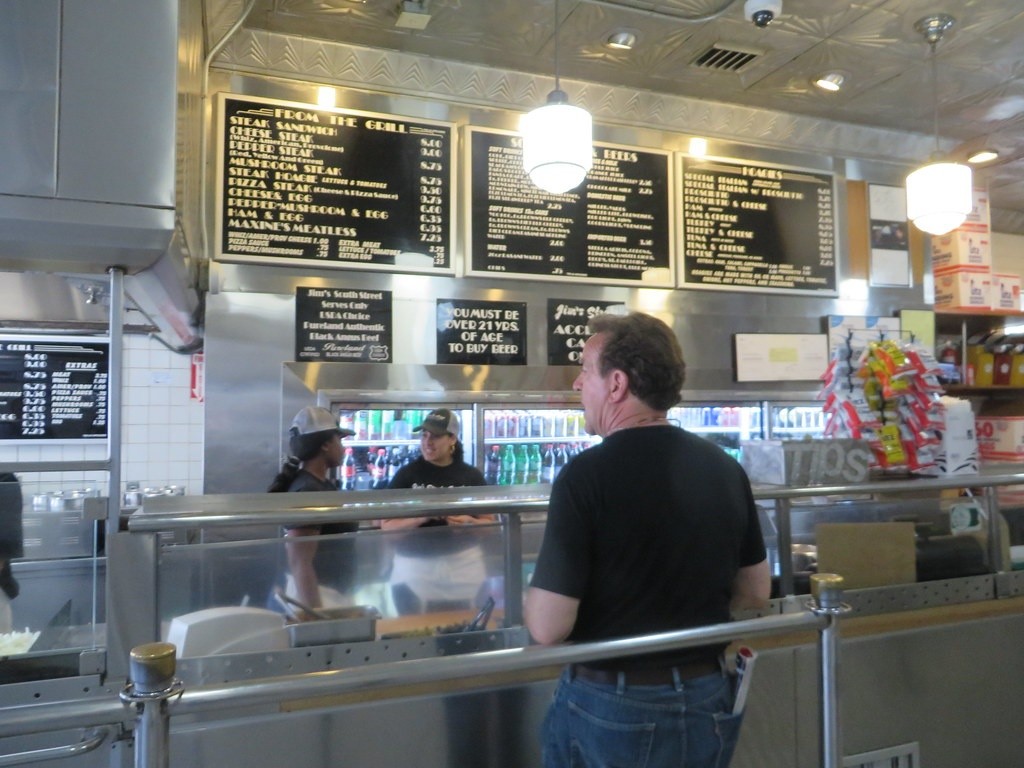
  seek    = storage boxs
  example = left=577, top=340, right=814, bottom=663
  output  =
left=739, top=439, right=869, bottom=487
left=930, top=191, right=1023, bottom=310
left=970, top=416, right=1024, bottom=509
left=966, top=345, right=1024, bottom=388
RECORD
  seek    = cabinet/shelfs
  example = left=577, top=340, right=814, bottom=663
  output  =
left=935, top=309, right=1024, bottom=390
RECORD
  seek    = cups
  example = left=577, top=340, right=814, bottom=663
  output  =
left=31, top=485, right=184, bottom=511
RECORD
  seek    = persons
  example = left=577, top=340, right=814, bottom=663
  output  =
left=0, top=470, right=25, bottom=636
left=265, top=406, right=360, bottom=626
left=523, top=311, right=770, bottom=768
left=378, top=408, right=504, bottom=618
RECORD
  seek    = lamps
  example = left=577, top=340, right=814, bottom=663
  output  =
left=600, top=27, right=642, bottom=51
left=519, top=0, right=593, bottom=196
left=904, top=13, right=973, bottom=237
left=810, top=69, right=851, bottom=92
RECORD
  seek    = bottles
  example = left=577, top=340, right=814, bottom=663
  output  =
left=337, top=410, right=604, bottom=491
left=666, top=407, right=829, bottom=464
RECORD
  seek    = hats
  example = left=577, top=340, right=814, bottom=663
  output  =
left=412, top=408, right=461, bottom=437
left=289, top=406, right=356, bottom=436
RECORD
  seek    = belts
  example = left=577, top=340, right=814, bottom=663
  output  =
left=573, top=652, right=725, bottom=685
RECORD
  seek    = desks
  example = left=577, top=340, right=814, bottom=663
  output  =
left=0, top=556, right=107, bottom=661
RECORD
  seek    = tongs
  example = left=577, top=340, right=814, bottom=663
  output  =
left=274, top=592, right=333, bottom=624
left=454, top=597, right=496, bottom=653
